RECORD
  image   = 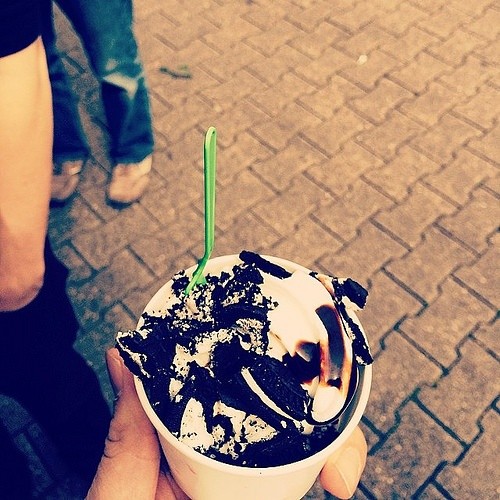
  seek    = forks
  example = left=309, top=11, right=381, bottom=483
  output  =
left=184, top=126, right=217, bottom=295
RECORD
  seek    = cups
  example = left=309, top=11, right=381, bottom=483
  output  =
left=133, top=254, right=373, bottom=500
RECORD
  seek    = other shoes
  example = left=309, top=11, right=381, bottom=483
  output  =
left=50, top=160, right=86, bottom=206
left=109, top=152, right=152, bottom=209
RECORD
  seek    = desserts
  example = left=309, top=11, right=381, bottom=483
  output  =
left=115, top=246, right=374, bottom=468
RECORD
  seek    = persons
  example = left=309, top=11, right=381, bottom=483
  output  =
left=0, top=1, right=118, bottom=500
left=32, top=0, right=155, bottom=209
left=86, top=344, right=370, bottom=500
